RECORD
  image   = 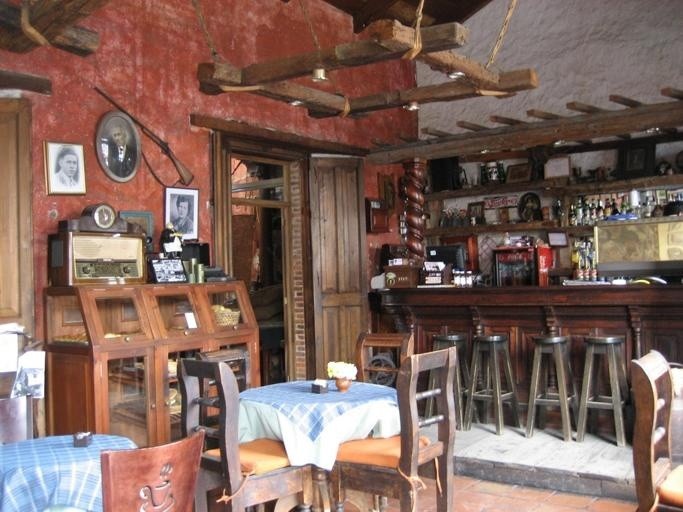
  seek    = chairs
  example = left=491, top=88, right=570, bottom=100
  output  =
left=355, top=329, right=415, bottom=382
left=101, top=428, right=204, bottom=512
left=316, top=344, right=457, bottom=512
left=177, top=357, right=312, bottom=512
left=630, top=349, right=683, bottom=512
left=0, top=393, right=35, bottom=442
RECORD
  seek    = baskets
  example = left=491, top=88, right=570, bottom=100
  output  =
left=215, top=311, right=241, bottom=327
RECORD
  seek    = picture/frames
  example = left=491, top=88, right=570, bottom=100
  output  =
left=505, top=162, right=532, bottom=183
left=43, top=140, right=87, bottom=196
left=150, top=257, right=188, bottom=283
left=546, top=229, right=570, bottom=248
left=467, top=201, right=486, bottom=218
left=163, top=186, right=199, bottom=242
left=439, top=234, right=480, bottom=274
left=94, top=110, right=142, bottom=183
left=378, top=171, right=398, bottom=213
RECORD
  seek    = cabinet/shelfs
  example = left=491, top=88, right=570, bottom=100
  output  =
left=44, top=281, right=260, bottom=448
left=427, top=174, right=683, bottom=276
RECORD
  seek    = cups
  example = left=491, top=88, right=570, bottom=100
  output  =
left=572, top=167, right=581, bottom=176
left=542, top=207, right=549, bottom=219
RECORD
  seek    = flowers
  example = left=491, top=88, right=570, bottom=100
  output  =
left=327, top=360, right=358, bottom=380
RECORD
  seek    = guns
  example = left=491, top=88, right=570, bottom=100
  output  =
left=95, top=86, right=193, bottom=186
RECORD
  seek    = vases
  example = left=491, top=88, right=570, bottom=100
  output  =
left=336, top=377, right=352, bottom=393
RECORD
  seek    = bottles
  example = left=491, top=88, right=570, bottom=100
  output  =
left=196, top=264, right=204, bottom=283
left=498, top=161, right=504, bottom=183
left=190, top=258, right=197, bottom=283
left=454, top=271, right=473, bottom=289
left=439, top=208, right=476, bottom=226
left=570, top=240, right=598, bottom=281
left=502, top=254, right=530, bottom=285
left=504, top=232, right=511, bottom=246
left=480, top=164, right=487, bottom=185
left=556, top=190, right=683, bottom=226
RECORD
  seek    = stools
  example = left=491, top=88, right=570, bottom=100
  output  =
left=523, top=335, right=583, bottom=441
left=423, top=330, right=481, bottom=427
left=462, top=333, right=523, bottom=435
left=576, top=334, right=635, bottom=447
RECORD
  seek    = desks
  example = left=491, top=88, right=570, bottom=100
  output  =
left=0, top=433, right=139, bottom=512
left=235, top=378, right=401, bottom=512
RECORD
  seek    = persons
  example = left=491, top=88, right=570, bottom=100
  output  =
left=54, top=146, right=80, bottom=186
left=168, top=194, right=194, bottom=233
left=522, top=196, right=539, bottom=221
left=103, top=118, right=137, bottom=177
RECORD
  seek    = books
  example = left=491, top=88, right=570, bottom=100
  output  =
left=204, top=265, right=231, bottom=283
